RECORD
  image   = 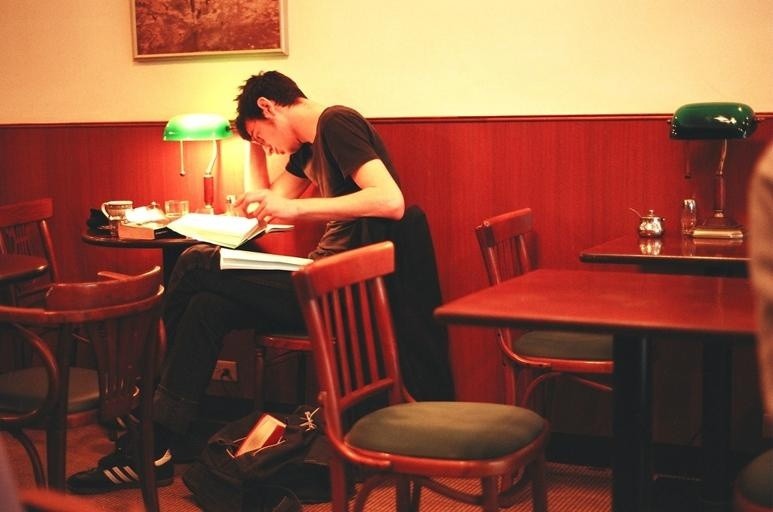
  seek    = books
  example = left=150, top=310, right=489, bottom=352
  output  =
left=168, top=213, right=295, bottom=249
left=219, top=249, right=315, bottom=271
left=117, top=214, right=179, bottom=240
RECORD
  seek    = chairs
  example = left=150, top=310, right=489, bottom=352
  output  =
left=288, top=241, right=551, bottom=512
left=0, top=265, right=168, bottom=512
left=0, top=197, right=64, bottom=368
left=255, top=206, right=421, bottom=418
left=476, top=207, right=615, bottom=510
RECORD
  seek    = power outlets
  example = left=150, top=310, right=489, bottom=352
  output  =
left=211, top=361, right=238, bottom=383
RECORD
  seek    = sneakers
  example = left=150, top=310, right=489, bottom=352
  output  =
left=69, top=448, right=173, bottom=493
left=96, top=411, right=144, bottom=438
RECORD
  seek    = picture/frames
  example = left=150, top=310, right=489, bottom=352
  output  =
left=128, top=0, right=289, bottom=62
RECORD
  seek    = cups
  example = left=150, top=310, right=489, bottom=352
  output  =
left=680, top=199, right=697, bottom=236
left=101, top=200, right=134, bottom=237
left=165, top=200, right=189, bottom=216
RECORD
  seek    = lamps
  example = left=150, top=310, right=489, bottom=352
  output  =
left=163, top=112, right=233, bottom=215
left=668, top=102, right=757, bottom=239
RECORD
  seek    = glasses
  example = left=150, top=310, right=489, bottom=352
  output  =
left=249, top=120, right=262, bottom=145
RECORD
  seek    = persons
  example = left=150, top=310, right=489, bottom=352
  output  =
left=746, top=138, right=772, bottom=424
left=63, top=70, right=406, bottom=493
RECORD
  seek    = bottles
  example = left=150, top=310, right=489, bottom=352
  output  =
left=639, top=239, right=663, bottom=255
left=637, top=210, right=667, bottom=237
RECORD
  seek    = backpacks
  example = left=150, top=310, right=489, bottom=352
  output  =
left=183, top=403, right=350, bottom=511
